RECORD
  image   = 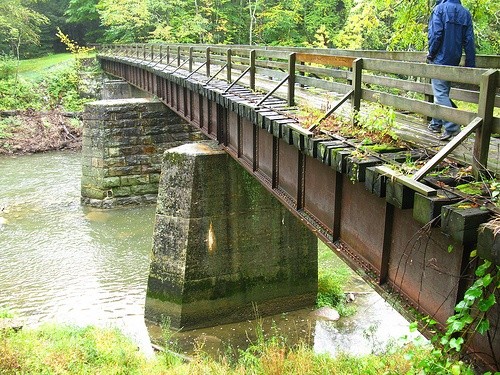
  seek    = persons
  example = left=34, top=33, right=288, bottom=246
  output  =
left=426, top=0, right=477, bottom=141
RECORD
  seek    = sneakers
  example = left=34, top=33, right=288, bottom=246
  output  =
left=427, top=124, right=442, bottom=133
left=437, top=129, right=460, bottom=140
